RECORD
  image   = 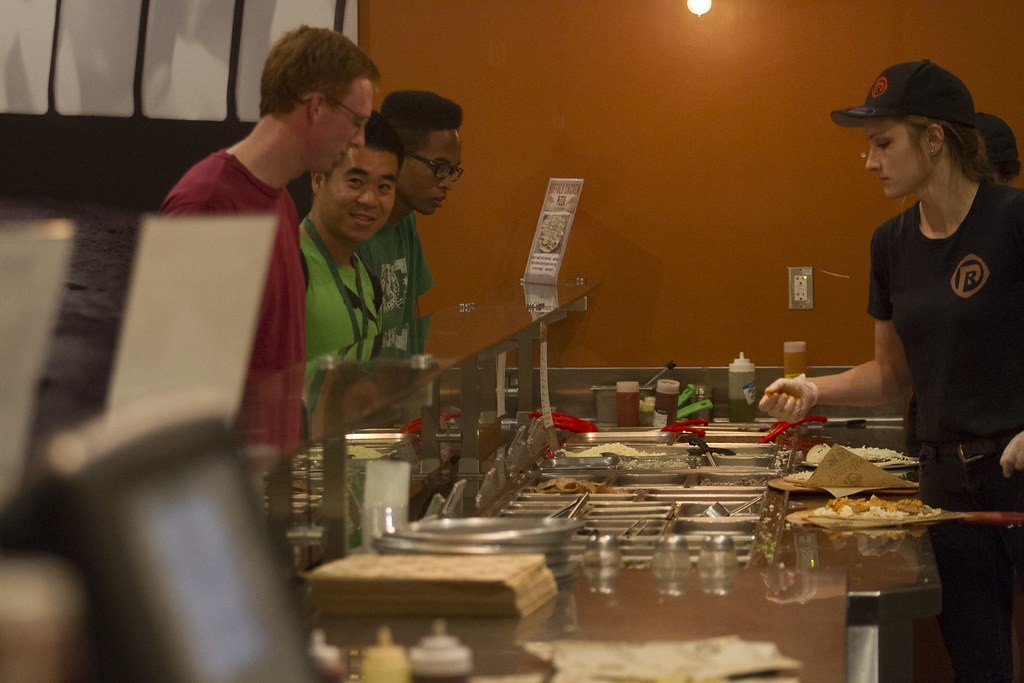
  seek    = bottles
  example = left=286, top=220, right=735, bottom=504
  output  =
left=408, top=617, right=474, bottom=683
left=728, top=351, right=756, bottom=423
left=651, top=536, right=691, bottom=597
left=616, top=381, right=641, bottom=427
left=584, top=534, right=622, bottom=595
left=696, top=534, right=736, bottom=596
left=783, top=340, right=807, bottom=379
left=307, top=627, right=344, bottom=683
left=652, top=379, right=680, bottom=427
left=358, top=625, right=413, bottom=683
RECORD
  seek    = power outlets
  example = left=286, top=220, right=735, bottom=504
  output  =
left=788, top=266, right=813, bottom=309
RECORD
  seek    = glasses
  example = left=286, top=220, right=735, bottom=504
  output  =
left=406, top=152, right=463, bottom=182
left=302, top=95, right=367, bottom=128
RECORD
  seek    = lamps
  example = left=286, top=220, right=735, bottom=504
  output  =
left=687, top=0, right=712, bottom=17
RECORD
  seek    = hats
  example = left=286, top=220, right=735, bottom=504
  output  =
left=830, top=58, right=975, bottom=128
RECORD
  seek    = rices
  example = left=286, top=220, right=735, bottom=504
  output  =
left=564, top=441, right=668, bottom=458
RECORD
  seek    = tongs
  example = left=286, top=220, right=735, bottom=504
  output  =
left=549, top=492, right=590, bottom=519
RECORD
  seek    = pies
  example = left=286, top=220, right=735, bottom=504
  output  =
left=812, top=493, right=942, bottom=520
left=805, top=443, right=919, bottom=466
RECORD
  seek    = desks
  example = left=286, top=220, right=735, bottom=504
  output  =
left=298, top=567, right=848, bottom=683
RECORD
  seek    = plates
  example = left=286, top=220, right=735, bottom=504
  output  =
left=372, top=514, right=588, bottom=587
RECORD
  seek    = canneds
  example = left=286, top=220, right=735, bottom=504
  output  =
left=616, top=381, right=639, bottom=427
left=652, top=379, right=679, bottom=427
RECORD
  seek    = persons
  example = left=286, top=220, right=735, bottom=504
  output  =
left=159, top=25, right=463, bottom=584
left=759, top=59, right=1024, bottom=683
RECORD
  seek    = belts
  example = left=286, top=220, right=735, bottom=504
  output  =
left=923, top=436, right=1007, bottom=463
